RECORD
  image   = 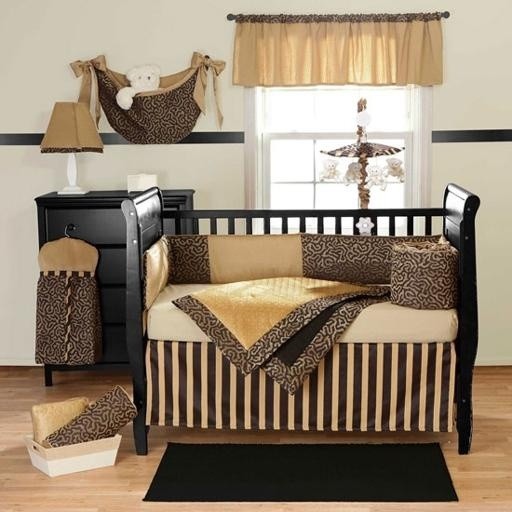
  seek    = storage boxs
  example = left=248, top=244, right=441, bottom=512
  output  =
left=23, top=434, right=124, bottom=479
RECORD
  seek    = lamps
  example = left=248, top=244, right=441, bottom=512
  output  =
left=40, top=102, right=105, bottom=195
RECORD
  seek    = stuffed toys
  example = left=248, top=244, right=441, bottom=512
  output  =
left=115, top=64, right=165, bottom=111
left=317, top=155, right=406, bottom=190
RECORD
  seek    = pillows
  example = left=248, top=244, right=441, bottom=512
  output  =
left=143, top=235, right=171, bottom=309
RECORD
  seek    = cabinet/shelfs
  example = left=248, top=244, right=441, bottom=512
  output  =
left=34, top=190, right=195, bottom=387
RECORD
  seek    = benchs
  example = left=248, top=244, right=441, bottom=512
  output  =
left=121, top=182, right=482, bottom=456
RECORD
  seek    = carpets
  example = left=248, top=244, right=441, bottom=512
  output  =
left=142, top=441, right=459, bottom=503
